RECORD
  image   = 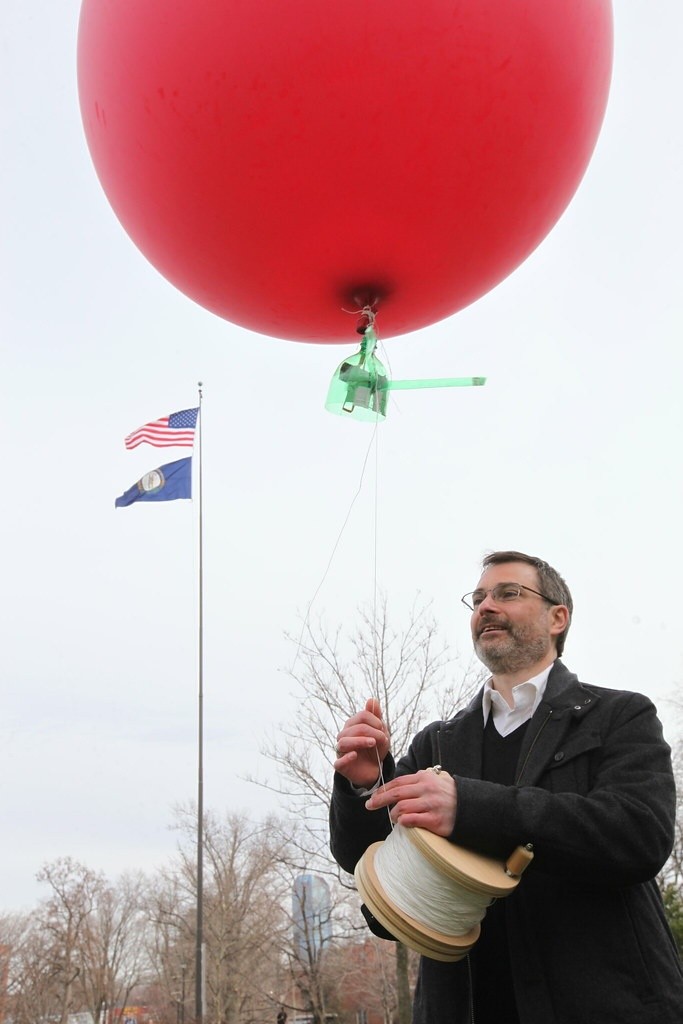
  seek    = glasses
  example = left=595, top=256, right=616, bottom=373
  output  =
left=460, top=582, right=558, bottom=611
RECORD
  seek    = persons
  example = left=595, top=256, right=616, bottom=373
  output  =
left=328, top=550, right=683, bottom=1023
left=275, top=1007, right=287, bottom=1023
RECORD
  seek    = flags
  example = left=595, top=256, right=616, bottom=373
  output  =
left=114, top=456, right=192, bottom=508
left=124, top=406, right=199, bottom=450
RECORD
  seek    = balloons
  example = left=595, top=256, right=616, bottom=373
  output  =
left=76, top=0, right=615, bottom=346
